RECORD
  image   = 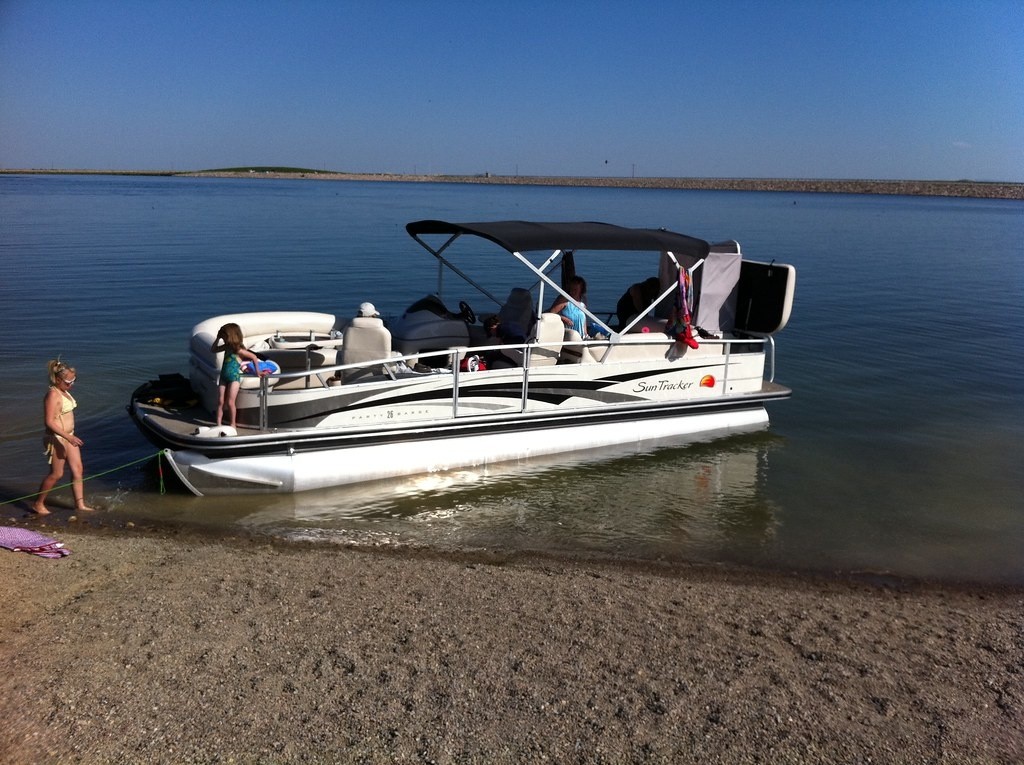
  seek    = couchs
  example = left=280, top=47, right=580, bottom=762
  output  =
left=191, top=310, right=336, bottom=390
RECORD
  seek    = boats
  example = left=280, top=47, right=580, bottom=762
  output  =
left=123, top=219, right=800, bottom=495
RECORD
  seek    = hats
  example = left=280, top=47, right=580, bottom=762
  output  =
left=357, top=301, right=380, bottom=317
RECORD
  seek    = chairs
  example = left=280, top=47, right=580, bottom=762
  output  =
left=342, top=317, right=392, bottom=382
left=493, top=312, right=566, bottom=368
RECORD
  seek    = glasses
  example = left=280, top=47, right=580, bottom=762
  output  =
left=59, top=376, right=77, bottom=385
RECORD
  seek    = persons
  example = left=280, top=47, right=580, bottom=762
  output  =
left=483, top=313, right=536, bottom=365
left=617, top=276, right=661, bottom=332
left=211, top=324, right=271, bottom=435
left=544, top=276, right=595, bottom=341
left=356, top=301, right=383, bottom=318
left=32, top=358, right=99, bottom=514
